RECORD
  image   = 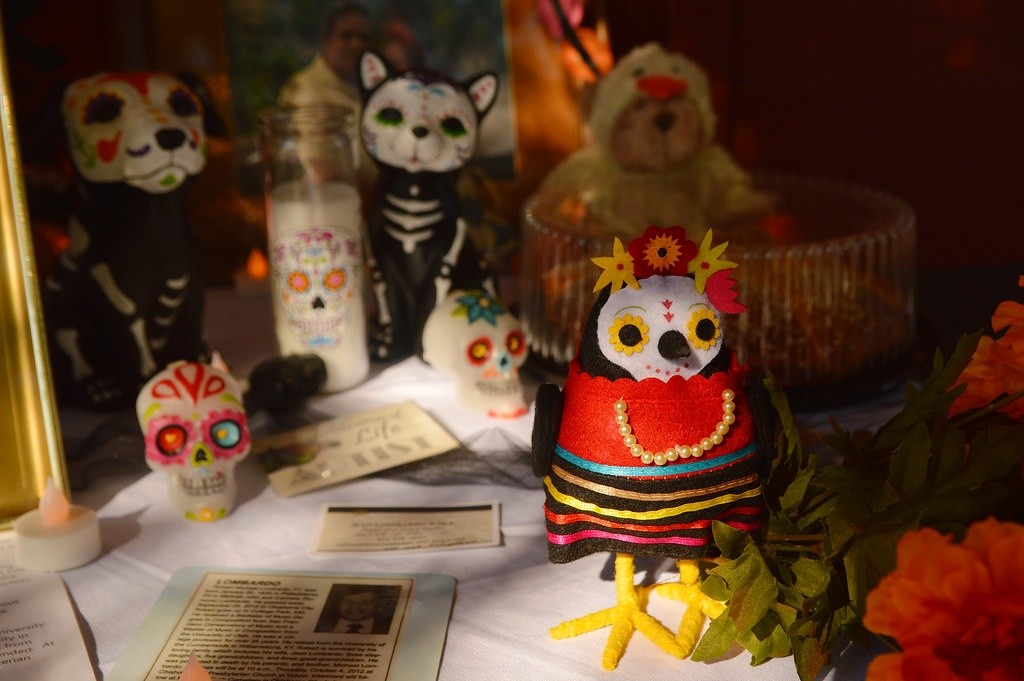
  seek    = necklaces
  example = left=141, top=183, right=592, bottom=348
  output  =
left=614, top=389, right=735, bottom=465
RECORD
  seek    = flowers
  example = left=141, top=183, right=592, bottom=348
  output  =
left=590, top=222, right=1024, bottom=681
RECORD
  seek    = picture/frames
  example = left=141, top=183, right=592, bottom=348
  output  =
left=120, top=0, right=587, bottom=290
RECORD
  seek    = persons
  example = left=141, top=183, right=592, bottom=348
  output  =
left=316, top=584, right=393, bottom=635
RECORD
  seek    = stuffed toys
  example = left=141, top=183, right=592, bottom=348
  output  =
left=528, top=43, right=785, bottom=246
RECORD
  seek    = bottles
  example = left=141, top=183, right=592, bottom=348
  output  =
left=264, top=103, right=369, bottom=392
